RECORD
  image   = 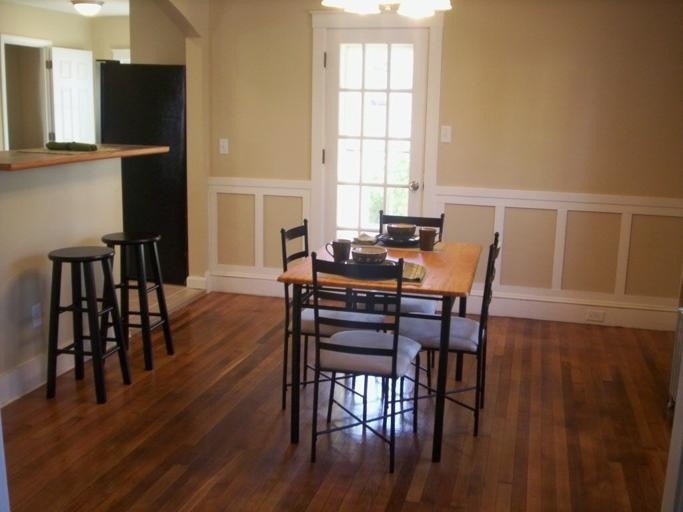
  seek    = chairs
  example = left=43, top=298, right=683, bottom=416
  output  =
left=381, top=229, right=503, bottom=446
left=353, top=207, right=447, bottom=396
left=307, top=250, right=422, bottom=476
left=276, top=219, right=374, bottom=418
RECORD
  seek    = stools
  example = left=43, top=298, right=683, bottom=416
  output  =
left=98, top=230, right=175, bottom=372
left=40, top=244, right=133, bottom=406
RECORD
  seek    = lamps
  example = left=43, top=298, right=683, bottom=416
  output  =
left=69, top=0, right=107, bottom=18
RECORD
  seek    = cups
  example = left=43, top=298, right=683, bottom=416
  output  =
left=418, top=227, right=442, bottom=251
left=325, top=240, right=350, bottom=261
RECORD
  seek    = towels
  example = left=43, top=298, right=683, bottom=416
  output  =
left=397, top=259, right=426, bottom=283
left=352, top=231, right=375, bottom=247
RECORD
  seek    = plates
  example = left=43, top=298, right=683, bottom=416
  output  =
left=347, top=260, right=397, bottom=269
left=376, top=233, right=419, bottom=246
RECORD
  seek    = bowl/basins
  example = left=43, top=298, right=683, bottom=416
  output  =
left=352, top=246, right=388, bottom=265
left=386, top=223, right=417, bottom=240
